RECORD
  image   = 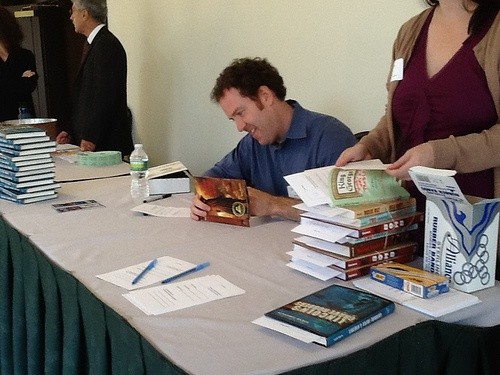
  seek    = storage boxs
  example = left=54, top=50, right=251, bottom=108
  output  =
left=409, top=166, right=500, bottom=294
left=145, top=161, right=192, bottom=195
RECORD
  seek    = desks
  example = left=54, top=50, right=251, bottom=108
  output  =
left=0, top=145, right=500, bottom=375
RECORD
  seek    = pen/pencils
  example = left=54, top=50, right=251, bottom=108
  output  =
left=143, top=193, right=172, bottom=203
left=162, top=262, right=210, bottom=284
left=132, top=259, right=157, bottom=284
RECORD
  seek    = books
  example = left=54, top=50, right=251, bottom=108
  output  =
left=283, top=159, right=425, bottom=283
left=193, top=175, right=271, bottom=228
left=251, top=283, right=395, bottom=348
left=0, top=128, right=62, bottom=205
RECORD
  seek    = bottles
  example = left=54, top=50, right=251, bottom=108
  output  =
left=129, top=144, right=149, bottom=198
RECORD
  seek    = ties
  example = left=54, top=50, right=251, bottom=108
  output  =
left=79, top=41, right=91, bottom=66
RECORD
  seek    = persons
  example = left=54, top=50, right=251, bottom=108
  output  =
left=336, top=0, right=500, bottom=283
left=190, top=57, right=357, bottom=222
left=0, top=7, right=40, bottom=123
left=58, top=0, right=135, bottom=162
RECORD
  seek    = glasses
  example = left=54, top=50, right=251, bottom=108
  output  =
left=69, top=8, right=87, bottom=14
